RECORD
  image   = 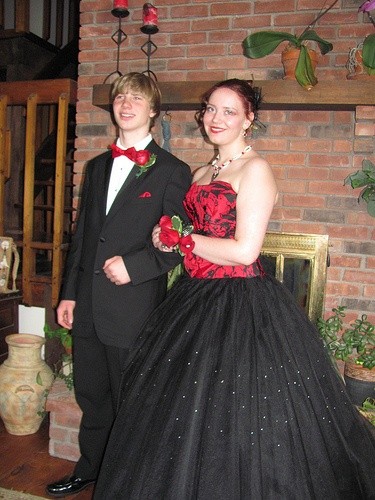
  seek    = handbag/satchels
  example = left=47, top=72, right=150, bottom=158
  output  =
left=0, top=237, right=19, bottom=294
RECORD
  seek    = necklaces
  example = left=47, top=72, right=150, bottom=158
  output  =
left=209, top=142, right=252, bottom=183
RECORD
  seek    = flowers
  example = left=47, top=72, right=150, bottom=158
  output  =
left=133, top=150, right=157, bottom=180
left=357, top=0, right=375, bottom=74
left=159, top=214, right=196, bottom=257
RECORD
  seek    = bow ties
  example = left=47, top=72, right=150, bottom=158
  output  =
left=107, top=144, right=138, bottom=163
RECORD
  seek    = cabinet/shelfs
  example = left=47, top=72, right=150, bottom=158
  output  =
left=0, top=291, right=23, bottom=366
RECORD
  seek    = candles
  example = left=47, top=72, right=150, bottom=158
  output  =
left=142, top=4, right=158, bottom=26
left=113, top=0, right=128, bottom=10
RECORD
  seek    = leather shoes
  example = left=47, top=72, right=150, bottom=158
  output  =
left=47, top=476, right=96, bottom=495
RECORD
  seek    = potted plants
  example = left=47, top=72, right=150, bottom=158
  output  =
left=245, top=0, right=338, bottom=91
left=346, top=42, right=375, bottom=79
left=36, top=324, right=74, bottom=418
left=316, top=305, right=374, bottom=407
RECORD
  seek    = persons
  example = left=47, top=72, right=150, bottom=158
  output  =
left=43, top=69, right=197, bottom=498
left=91, top=75, right=375, bottom=500
